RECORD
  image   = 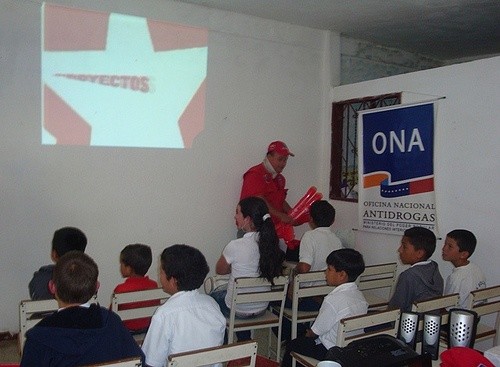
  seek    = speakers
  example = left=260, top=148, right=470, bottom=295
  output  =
left=448, top=308, right=478, bottom=350
left=395, top=311, right=419, bottom=353
left=422, top=310, right=442, bottom=361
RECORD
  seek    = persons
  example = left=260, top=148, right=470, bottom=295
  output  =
left=281, top=248, right=368, bottom=367
left=140, top=244, right=226, bottom=367
left=28, top=227, right=87, bottom=319
left=442, top=230, right=487, bottom=333
left=237, top=141, right=295, bottom=238
left=365, top=226, right=444, bottom=342
left=272, top=200, right=343, bottom=345
left=21, top=251, right=146, bottom=367
left=110, top=244, right=160, bottom=335
left=211, top=197, right=282, bottom=345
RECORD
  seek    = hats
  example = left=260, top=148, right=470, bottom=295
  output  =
left=268, top=141, right=295, bottom=157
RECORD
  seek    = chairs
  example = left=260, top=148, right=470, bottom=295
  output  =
left=18, top=246, right=500, bottom=367
